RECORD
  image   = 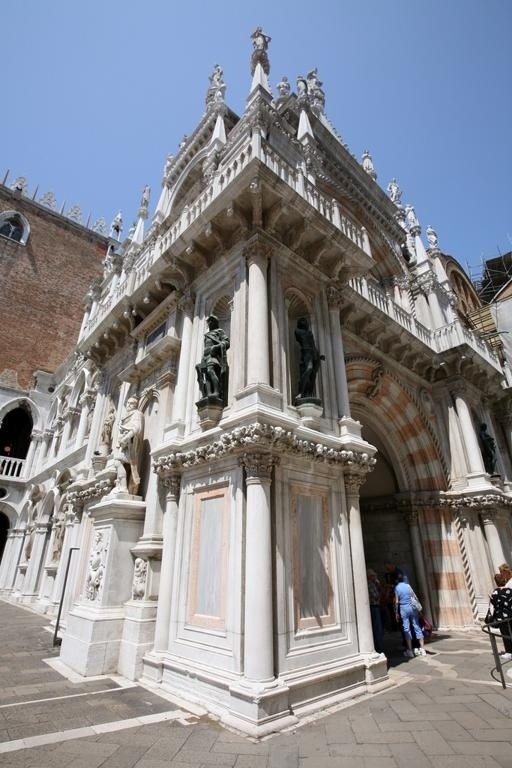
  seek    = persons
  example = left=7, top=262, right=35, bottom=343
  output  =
left=480, top=423, right=496, bottom=474
left=498, top=565, right=512, bottom=586
left=50, top=504, right=68, bottom=560
left=195, top=315, right=231, bottom=398
left=101, top=407, right=115, bottom=444
left=295, top=317, right=321, bottom=400
left=116, top=398, right=144, bottom=493
left=367, top=559, right=426, bottom=657
left=485, top=574, right=512, bottom=653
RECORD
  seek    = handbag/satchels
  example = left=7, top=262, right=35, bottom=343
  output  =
left=419, top=615, right=432, bottom=630
left=484, top=610, right=502, bottom=627
left=411, top=598, right=423, bottom=611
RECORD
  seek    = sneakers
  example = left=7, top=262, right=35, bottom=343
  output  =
left=402, top=646, right=428, bottom=658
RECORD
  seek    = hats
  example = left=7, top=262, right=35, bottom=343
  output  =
left=367, top=568, right=378, bottom=577
